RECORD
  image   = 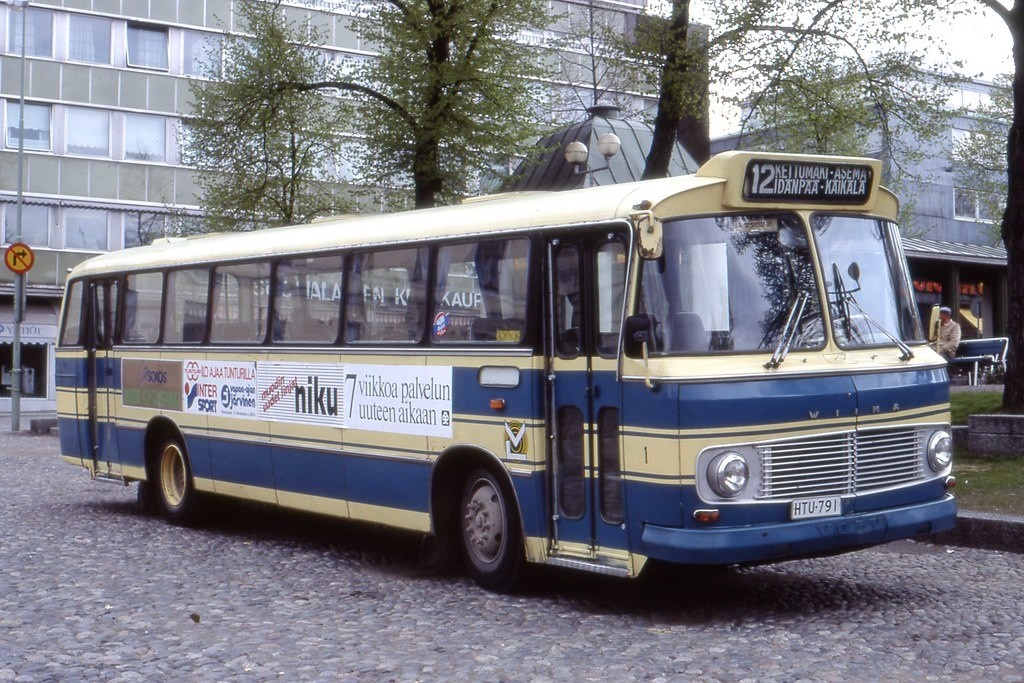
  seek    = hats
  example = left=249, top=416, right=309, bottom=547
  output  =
left=940, top=307, right=951, bottom=315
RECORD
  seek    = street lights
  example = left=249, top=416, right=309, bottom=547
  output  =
left=565, top=133, right=622, bottom=187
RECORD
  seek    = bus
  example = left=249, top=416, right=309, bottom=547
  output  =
left=55, top=147, right=962, bottom=595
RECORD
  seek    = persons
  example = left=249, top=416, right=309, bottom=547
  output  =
left=929, top=306, right=961, bottom=384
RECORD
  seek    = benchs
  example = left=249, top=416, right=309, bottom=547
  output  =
left=945, top=337, right=1009, bottom=385
left=147, top=317, right=525, bottom=343
